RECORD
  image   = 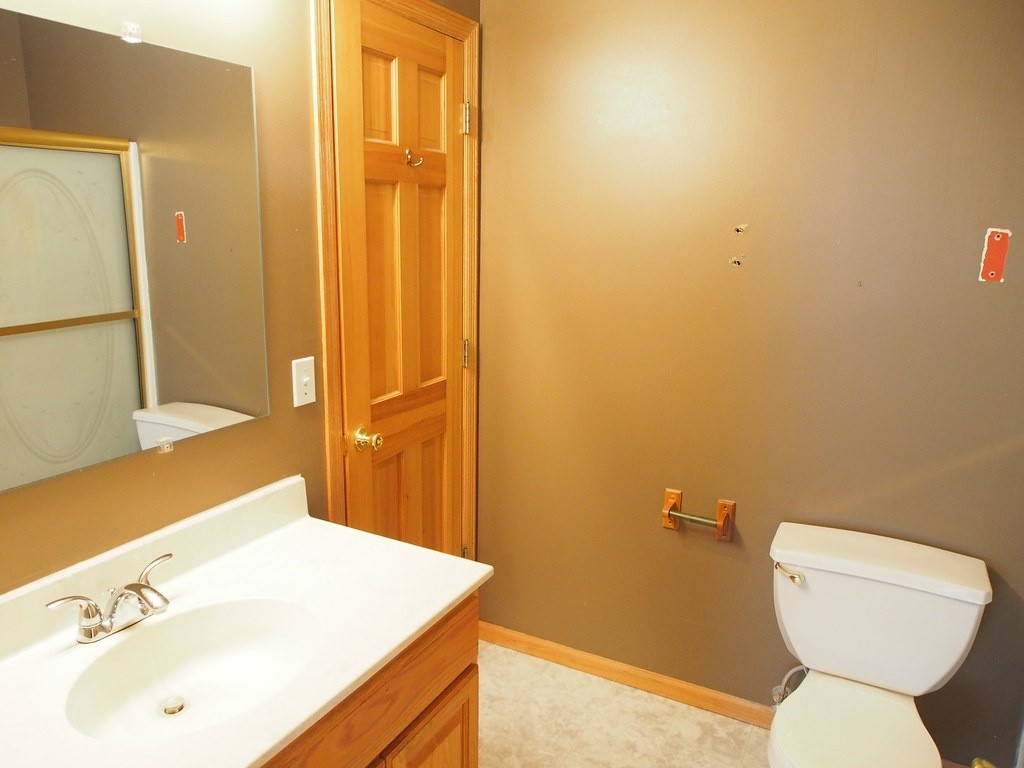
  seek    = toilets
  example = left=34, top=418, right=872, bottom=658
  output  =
left=131, top=400, right=257, bottom=451
left=761, top=520, right=994, bottom=768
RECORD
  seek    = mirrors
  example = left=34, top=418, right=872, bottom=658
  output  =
left=0, top=6, right=268, bottom=492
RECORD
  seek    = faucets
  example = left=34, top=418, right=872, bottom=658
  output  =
left=100, top=581, right=170, bottom=633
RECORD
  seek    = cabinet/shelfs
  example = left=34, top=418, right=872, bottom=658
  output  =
left=263, top=590, right=481, bottom=768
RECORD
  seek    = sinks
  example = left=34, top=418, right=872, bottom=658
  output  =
left=60, top=597, right=364, bottom=760
